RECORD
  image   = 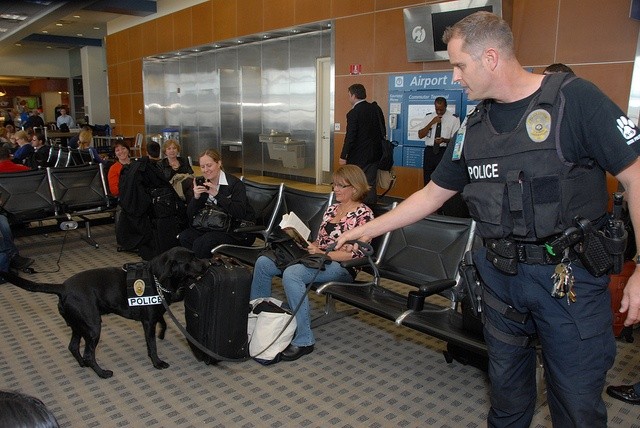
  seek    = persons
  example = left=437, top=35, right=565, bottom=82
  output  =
left=0, top=98, right=50, bottom=170
left=0, top=390, right=60, bottom=428
left=78, top=130, right=106, bottom=164
left=70, top=126, right=92, bottom=146
left=179, top=150, right=255, bottom=271
left=145, top=141, right=161, bottom=164
left=419, top=96, right=460, bottom=186
left=324, top=11, right=640, bottom=427
left=57, top=107, right=74, bottom=129
left=107, top=138, right=134, bottom=196
left=0, top=215, right=33, bottom=282
left=251, top=165, right=375, bottom=360
left=338, top=84, right=387, bottom=204
left=159, top=140, right=194, bottom=180
left=607, top=381, right=639, bottom=404
left=543, top=63, right=574, bottom=75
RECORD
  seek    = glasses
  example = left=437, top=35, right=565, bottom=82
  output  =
left=330, top=182, right=351, bottom=189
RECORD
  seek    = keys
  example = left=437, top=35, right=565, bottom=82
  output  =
left=548, top=255, right=577, bottom=305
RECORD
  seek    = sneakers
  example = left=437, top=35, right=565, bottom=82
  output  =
left=9, top=255, right=34, bottom=269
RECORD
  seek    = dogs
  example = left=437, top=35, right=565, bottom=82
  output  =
left=1, top=246, right=209, bottom=379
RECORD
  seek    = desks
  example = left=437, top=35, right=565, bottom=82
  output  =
left=94, top=136, right=135, bottom=157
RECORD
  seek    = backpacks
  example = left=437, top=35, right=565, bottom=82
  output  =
left=150, top=181, right=177, bottom=217
left=26, top=152, right=39, bottom=168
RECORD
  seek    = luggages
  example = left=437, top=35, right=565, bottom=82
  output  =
left=608, top=260, right=635, bottom=344
left=184, top=253, right=253, bottom=366
left=150, top=216, right=178, bottom=255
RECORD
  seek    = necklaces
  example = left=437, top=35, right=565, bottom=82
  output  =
left=169, top=160, right=180, bottom=166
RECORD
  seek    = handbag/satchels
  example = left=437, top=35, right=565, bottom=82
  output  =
left=377, top=138, right=399, bottom=170
left=60, top=123, right=69, bottom=132
left=247, top=296, right=297, bottom=366
left=120, top=163, right=152, bottom=218
left=191, top=203, right=233, bottom=232
left=170, top=172, right=194, bottom=200
left=376, top=169, right=396, bottom=192
left=115, top=201, right=148, bottom=256
left=268, top=226, right=303, bottom=266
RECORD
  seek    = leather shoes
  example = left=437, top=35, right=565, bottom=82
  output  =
left=607, top=385, right=639, bottom=405
left=281, top=344, right=313, bottom=361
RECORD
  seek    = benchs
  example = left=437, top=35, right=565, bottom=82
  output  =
left=99, top=156, right=195, bottom=208
left=211, top=183, right=398, bottom=332
left=317, top=201, right=549, bottom=414
left=67, top=146, right=94, bottom=166
left=0, top=163, right=108, bottom=249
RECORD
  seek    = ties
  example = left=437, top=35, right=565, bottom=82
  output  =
left=432, top=117, right=443, bottom=156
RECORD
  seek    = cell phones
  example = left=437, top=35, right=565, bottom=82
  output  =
left=195, top=176, right=206, bottom=186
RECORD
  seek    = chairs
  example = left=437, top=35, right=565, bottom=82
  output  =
left=46, top=144, right=71, bottom=167
left=128, top=133, right=143, bottom=157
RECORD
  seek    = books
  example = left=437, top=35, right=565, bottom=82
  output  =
left=279, top=211, right=313, bottom=252
